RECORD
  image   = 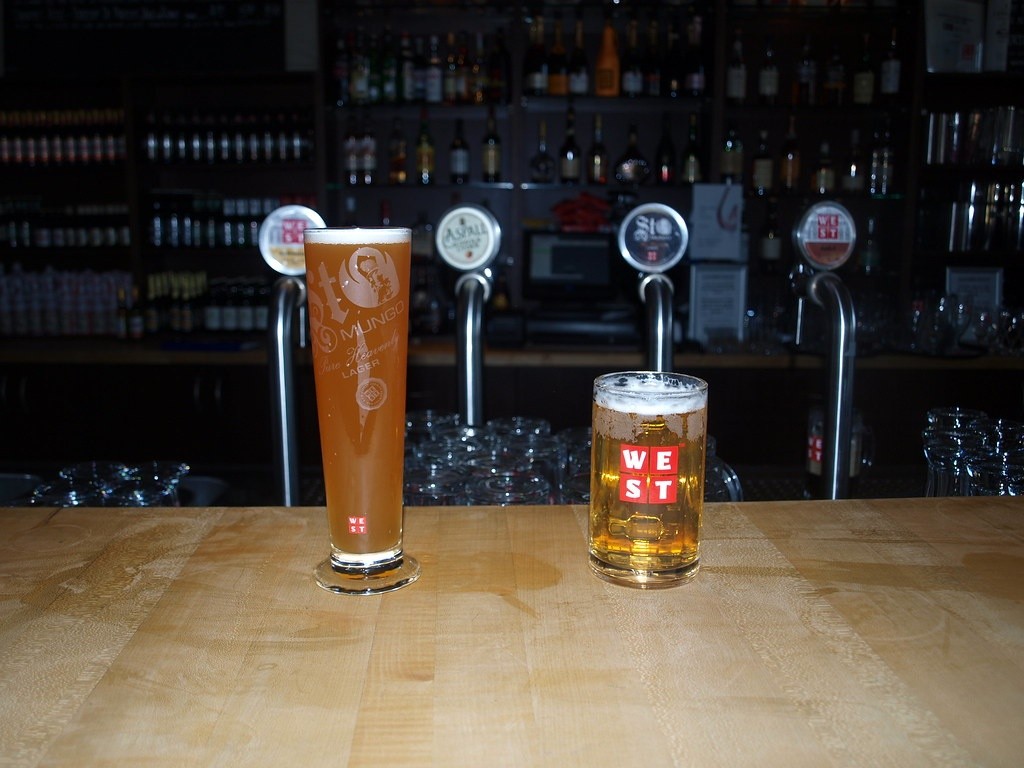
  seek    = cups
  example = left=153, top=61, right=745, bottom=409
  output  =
left=590, top=371, right=707, bottom=587
left=398, top=407, right=750, bottom=508
left=6, top=454, right=193, bottom=506
left=922, top=404, right=1023, bottom=495
left=304, top=226, right=421, bottom=598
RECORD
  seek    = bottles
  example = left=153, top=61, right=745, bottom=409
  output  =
left=1, top=2, right=899, bottom=342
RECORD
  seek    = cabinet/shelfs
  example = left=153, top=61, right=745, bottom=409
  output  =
left=0, top=0, right=1024, bottom=341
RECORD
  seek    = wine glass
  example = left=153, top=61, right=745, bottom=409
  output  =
left=829, top=287, right=1024, bottom=356
